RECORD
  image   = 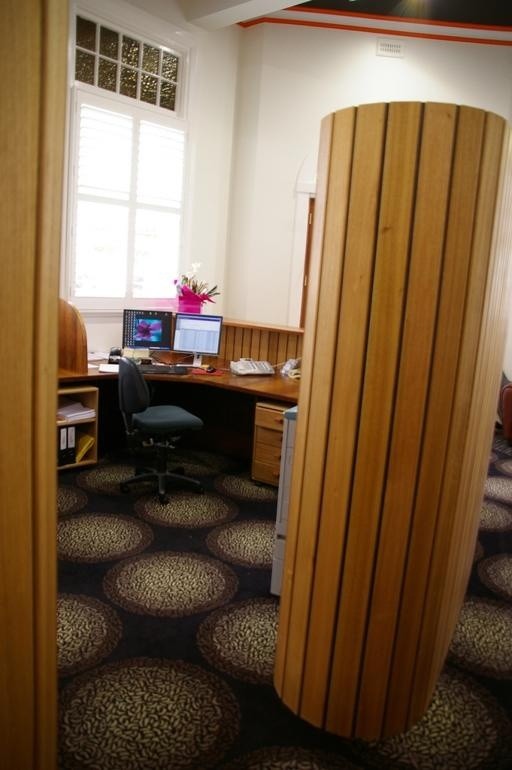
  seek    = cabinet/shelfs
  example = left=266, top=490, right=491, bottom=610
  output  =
left=250, top=398, right=289, bottom=488
left=56, top=386, right=100, bottom=471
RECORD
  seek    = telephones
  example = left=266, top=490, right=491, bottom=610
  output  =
left=230, top=358, right=275, bottom=376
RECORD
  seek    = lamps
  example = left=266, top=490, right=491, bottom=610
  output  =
left=293, top=155, right=319, bottom=328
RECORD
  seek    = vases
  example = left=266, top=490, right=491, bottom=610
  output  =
left=174, top=294, right=203, bottom=315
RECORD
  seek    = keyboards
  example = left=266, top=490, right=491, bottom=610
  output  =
left=136, top=364, right=187, bottom=375
left=99, top=363, right=119, bottom=374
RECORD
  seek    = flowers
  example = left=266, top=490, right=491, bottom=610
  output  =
left=173, top=261, right=221, bottom=301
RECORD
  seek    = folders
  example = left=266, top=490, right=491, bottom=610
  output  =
left=60, top=426, right=76, bottom=465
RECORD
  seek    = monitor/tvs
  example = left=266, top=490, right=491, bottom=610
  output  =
left=122, top=308, right=173, bottom=366
left=173, top=315, right=223, bottom=368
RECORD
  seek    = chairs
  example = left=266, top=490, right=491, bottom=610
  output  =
left=115, top=355, right=209, bottom=504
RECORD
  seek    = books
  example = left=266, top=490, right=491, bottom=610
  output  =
left=57, top=402, right=96, bottom=422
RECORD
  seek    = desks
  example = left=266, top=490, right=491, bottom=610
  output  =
left=58, top=351, right=302, bottom=485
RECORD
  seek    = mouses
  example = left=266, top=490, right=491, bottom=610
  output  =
left=205, top=367, right=216, bottom=373
left=141, top=359, right=152, bottom=365
